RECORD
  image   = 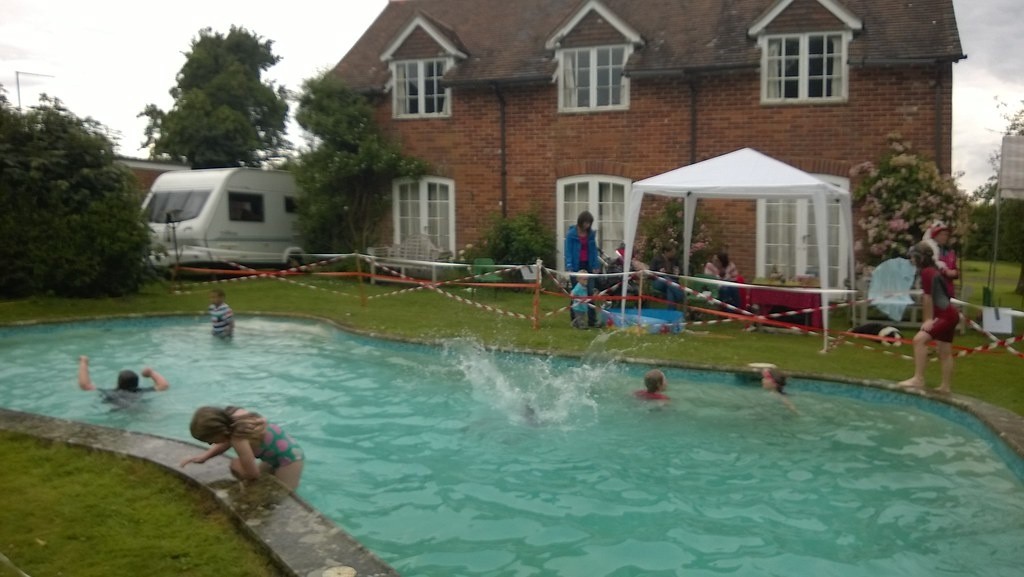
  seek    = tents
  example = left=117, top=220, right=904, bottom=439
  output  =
left=620, top=147, right=857, bottom=353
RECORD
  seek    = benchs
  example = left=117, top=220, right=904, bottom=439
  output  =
left=367, top=235, right=453, bottom=285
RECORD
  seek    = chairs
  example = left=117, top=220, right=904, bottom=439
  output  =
left=472, top=258, right=503, bottom=298
left=648, top=273, right=719, bottom=321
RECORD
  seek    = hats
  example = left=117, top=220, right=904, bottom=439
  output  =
left=931, top=226, right=953, bottom=239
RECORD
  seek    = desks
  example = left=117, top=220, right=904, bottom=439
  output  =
left=751, top=286, right=822, bottom=334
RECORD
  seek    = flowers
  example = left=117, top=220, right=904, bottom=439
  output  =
left=854, top=130, right=979, bottom=266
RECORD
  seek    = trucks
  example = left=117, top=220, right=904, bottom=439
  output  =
left=134, top=167, right=301, bottom=270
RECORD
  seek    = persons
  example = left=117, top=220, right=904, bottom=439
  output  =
left=208, top=289, right=234, bottom=333
left=704, top=251, right=741, bottom=314
left=79, top=355, right=168, bottom=391
left=181, top=406, right=305, bottom=490
left=760, top=367, right=787, bottom=393
left=570, top=270, right=599, bottom=330
left=644, top=369, right=668, bottom=393
left=931, top=225, right=959, bottom=299
left=608, top=242, right=640, bottom=309
left=648, top=243, right=684, bottom=311
left=564, top=211, right=602, bottom=328
left=899, top=241, right=960, bottom=392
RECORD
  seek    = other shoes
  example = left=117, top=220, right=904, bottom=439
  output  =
left=594, top=321, right=602, bottom=328
